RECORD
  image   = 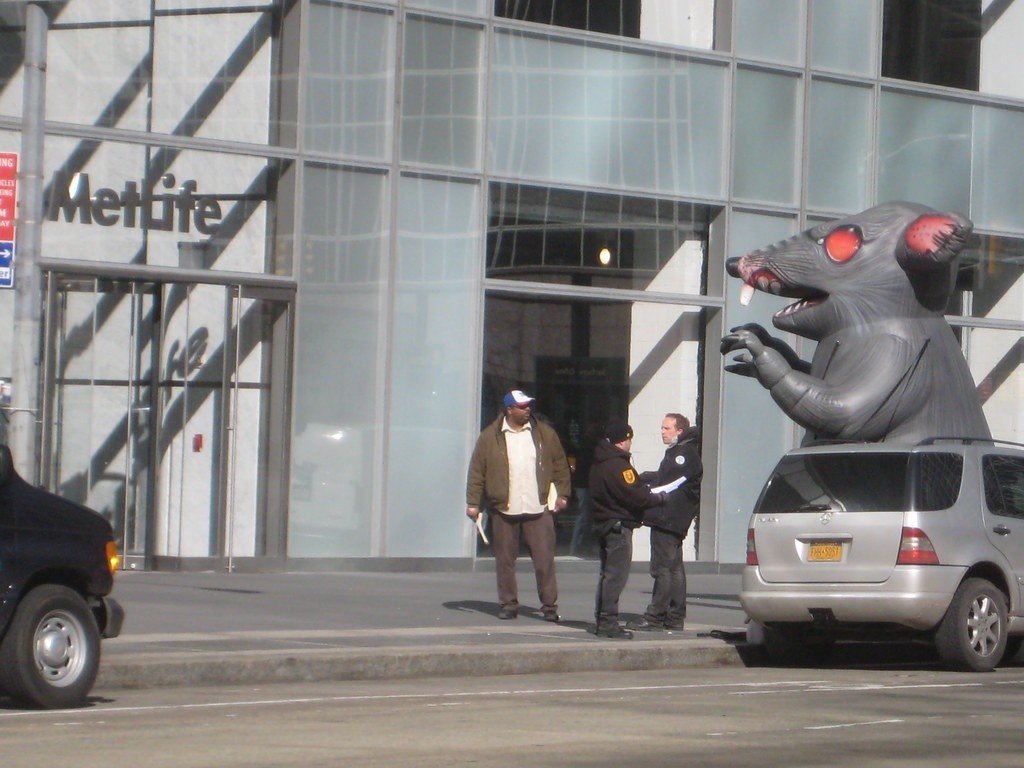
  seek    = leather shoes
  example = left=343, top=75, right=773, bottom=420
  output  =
left=663, top=620, right=685, bottom=633
left=626, top=618, right=663, bottom=632
left=596, top=628, right=633, bottom=639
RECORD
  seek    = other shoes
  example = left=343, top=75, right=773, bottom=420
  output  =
left=543, top=609, right=559, bottom=622
left=499, top=608, right=519, bottom=619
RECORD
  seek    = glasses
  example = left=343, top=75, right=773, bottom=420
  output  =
left=520, top=404, right=533, bottom=410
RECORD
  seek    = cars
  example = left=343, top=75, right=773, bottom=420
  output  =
left=738, top=434, right=1024, bottom=673
left=0, top=445, right=127, bottom=713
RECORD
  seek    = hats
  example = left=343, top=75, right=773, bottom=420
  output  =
left=606, top=423, right=633, bottom=443
left=503, top=390, right=536, bottom=408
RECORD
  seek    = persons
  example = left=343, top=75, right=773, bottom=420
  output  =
left=589, top=420, right=668, bottom=638
left=628, top=413, right=704, bottom=632
left=466, top=390, right=570, bottom=622
